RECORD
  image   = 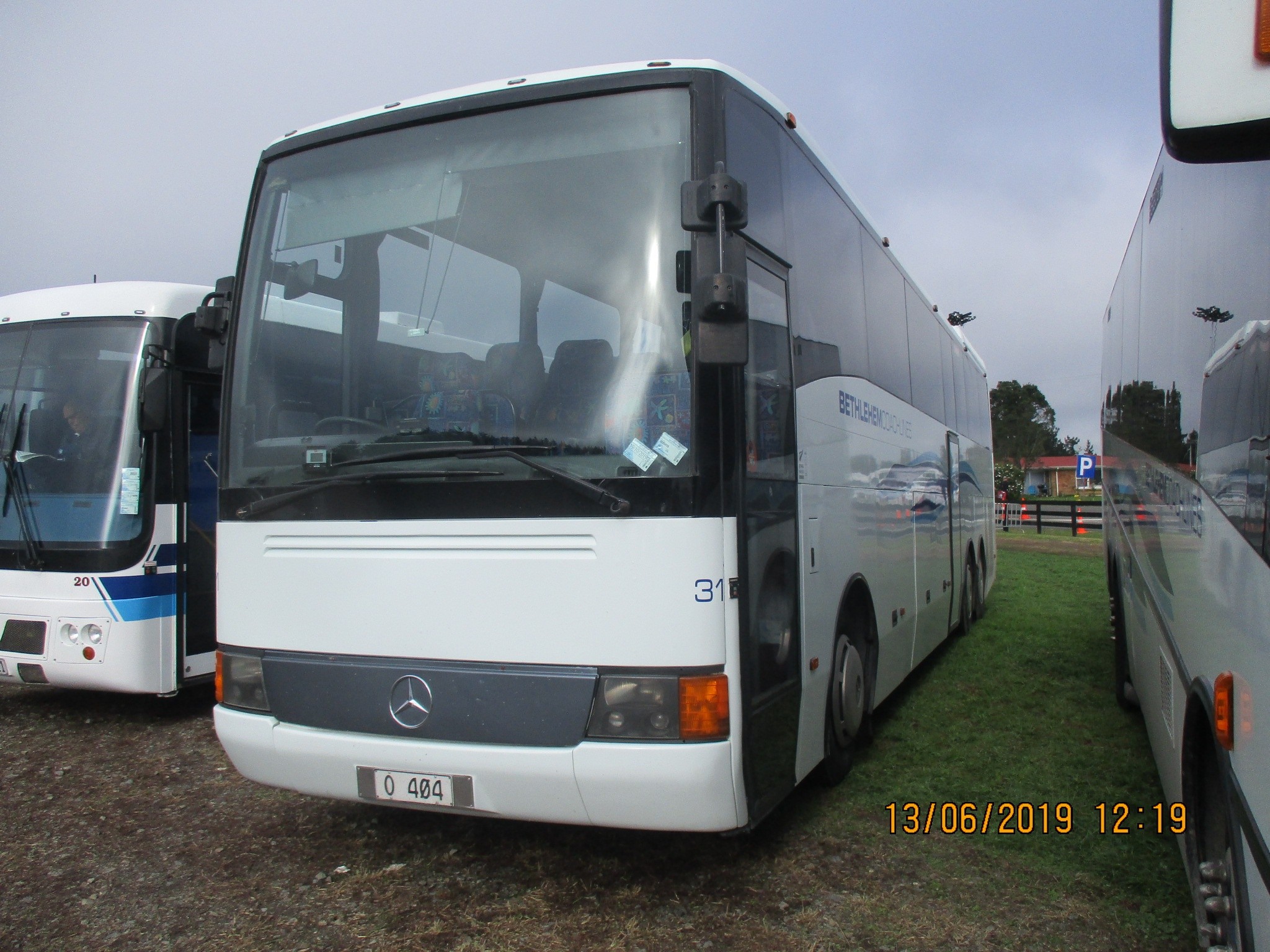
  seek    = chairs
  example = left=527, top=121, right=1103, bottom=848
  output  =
left=29, top=398, right=66, bottom=453
left=404, top=338, right=690, bottom=453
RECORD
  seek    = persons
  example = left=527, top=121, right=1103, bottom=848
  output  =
left=54, top=397, right=119, bottom=491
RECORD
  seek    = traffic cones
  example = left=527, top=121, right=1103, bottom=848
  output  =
left=1018, top=498, right=1031, bottom=520
left=1076, top=508, right=1093, bottom=534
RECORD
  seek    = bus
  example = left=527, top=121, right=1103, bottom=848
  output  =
left=1098, top=1, right=1268, bottom=952
left=1, top=280, right=510, bottom=700
left=168, top=60, right=997, bottom=837
